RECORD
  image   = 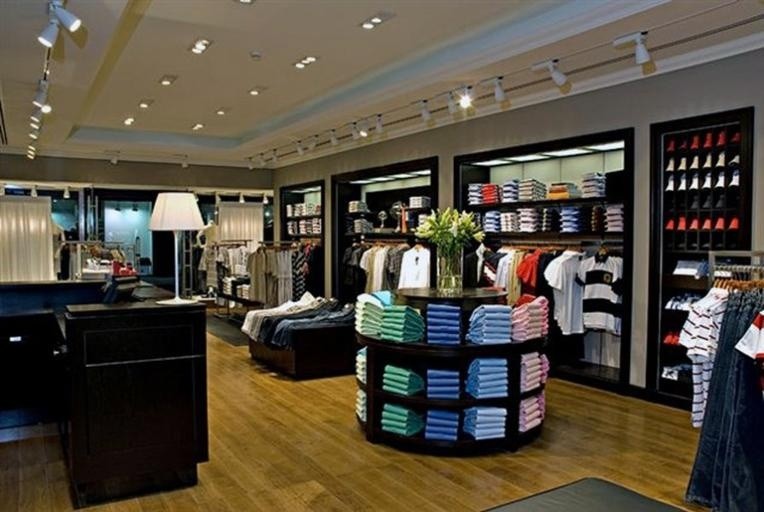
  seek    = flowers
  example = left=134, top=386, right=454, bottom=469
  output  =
left=411, top=206, right=485, bottom=282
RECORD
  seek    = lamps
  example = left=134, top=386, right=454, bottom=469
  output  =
left=182, top=159, right=189, bottom=168
left=111, top=151, right=121, bottom=165
left=26, top=79, right=49, bottom=162
left=53, top=6, right=82, bottom=33
left=37, top=23, right=61, bottom=49
left=149, top=191, right=204, bottom=305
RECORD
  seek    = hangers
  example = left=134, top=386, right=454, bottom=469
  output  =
left=358, top=238, right=408, bottom=253
left=712, top=263, right=764, bottom=294
left=505, top=238, right=609, bottom=253
left=256, top=243, right=294, bottom=257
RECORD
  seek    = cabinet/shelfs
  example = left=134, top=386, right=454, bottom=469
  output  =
left=351, top=285, right=550, bottom=457
left=285, top=211, right=322, bottom=238
left=345, top=205, right=432, bottom=239
left=646, top=106, right=754, bottom=400
left=466, top=198, right=624, bottom=240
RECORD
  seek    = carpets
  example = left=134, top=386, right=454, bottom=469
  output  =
left=207, top=311, right=251, bottom=346
left=484, top=475, right=685, bottom=512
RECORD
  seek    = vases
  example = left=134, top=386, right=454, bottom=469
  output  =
left=437, top=246, right=463, bottom=295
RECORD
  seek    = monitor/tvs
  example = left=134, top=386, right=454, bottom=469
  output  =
left=102, top=273, right=140, bottom=306
